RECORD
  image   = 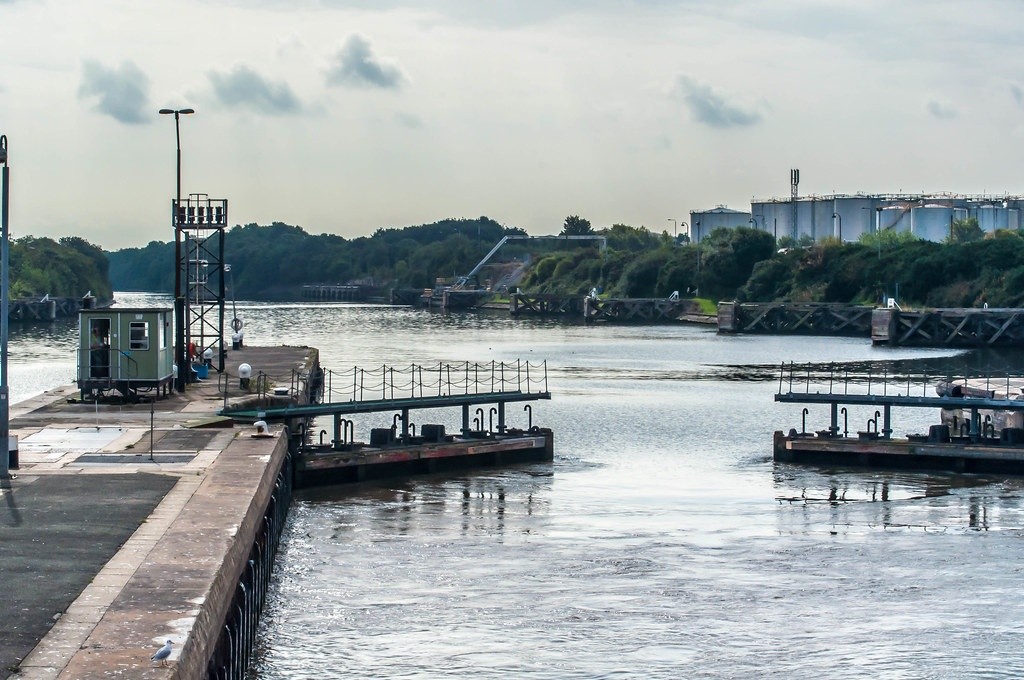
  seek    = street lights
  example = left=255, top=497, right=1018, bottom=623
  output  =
left=668, top=218, right=677, bottom=238
left=158, top=108, right=196, bottom=394
left=832, top=212, right=842, bottom=243
left=749, top=217, right=758, bottom=229
left=681, top=221, right=688, bottom=237
